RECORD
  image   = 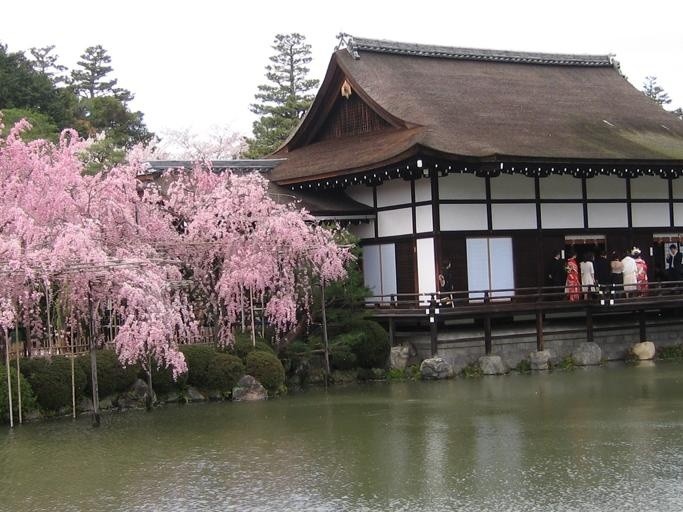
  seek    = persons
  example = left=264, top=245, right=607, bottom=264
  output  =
left=665, top=244, right=682, bottom=294
left=545, top=250, right=649, bottom=305
left=438, top=257, right=453, bottom=308
